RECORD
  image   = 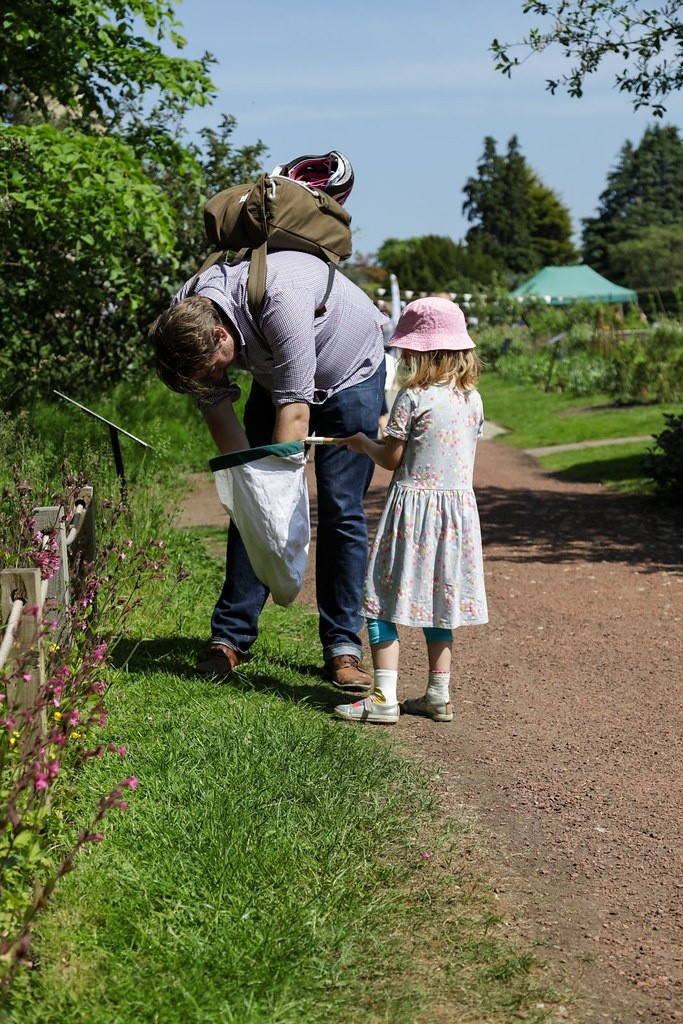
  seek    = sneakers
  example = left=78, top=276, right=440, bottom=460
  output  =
left=405, top=696, right=453, bottom=721
left=197, top=644, right=239, bottom=674
left=324, top=655, right=372, bottom=690
left=335, top=696, right=401, bottom=724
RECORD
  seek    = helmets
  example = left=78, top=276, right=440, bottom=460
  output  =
left=271, top=149, right=355, bottom=207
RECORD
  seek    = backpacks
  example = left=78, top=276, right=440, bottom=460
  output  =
left=191, top=177, right=355, bottom=314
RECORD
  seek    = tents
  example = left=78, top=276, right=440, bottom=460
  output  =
left=507, top=262, right=639, bottom=305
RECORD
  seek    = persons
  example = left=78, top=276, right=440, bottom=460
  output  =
left=148, top=248, right=387, bottom=689
left=333, top=296, right=489, bottom=726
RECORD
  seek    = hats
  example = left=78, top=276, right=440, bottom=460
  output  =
left=384, top=297, right=478, bottom=355
